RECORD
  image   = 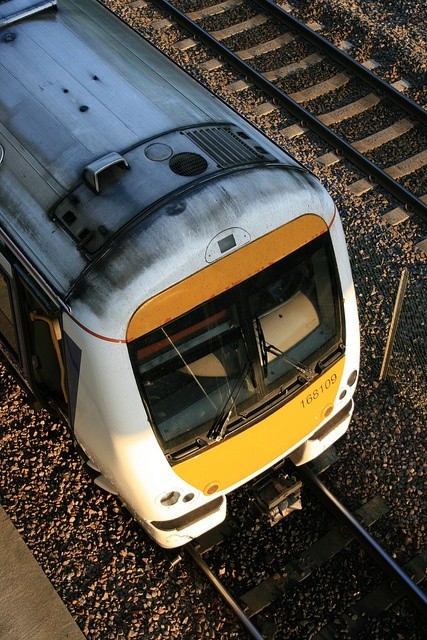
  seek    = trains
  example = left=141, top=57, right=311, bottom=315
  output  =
left=0, top=0, right=360, bottom=550
